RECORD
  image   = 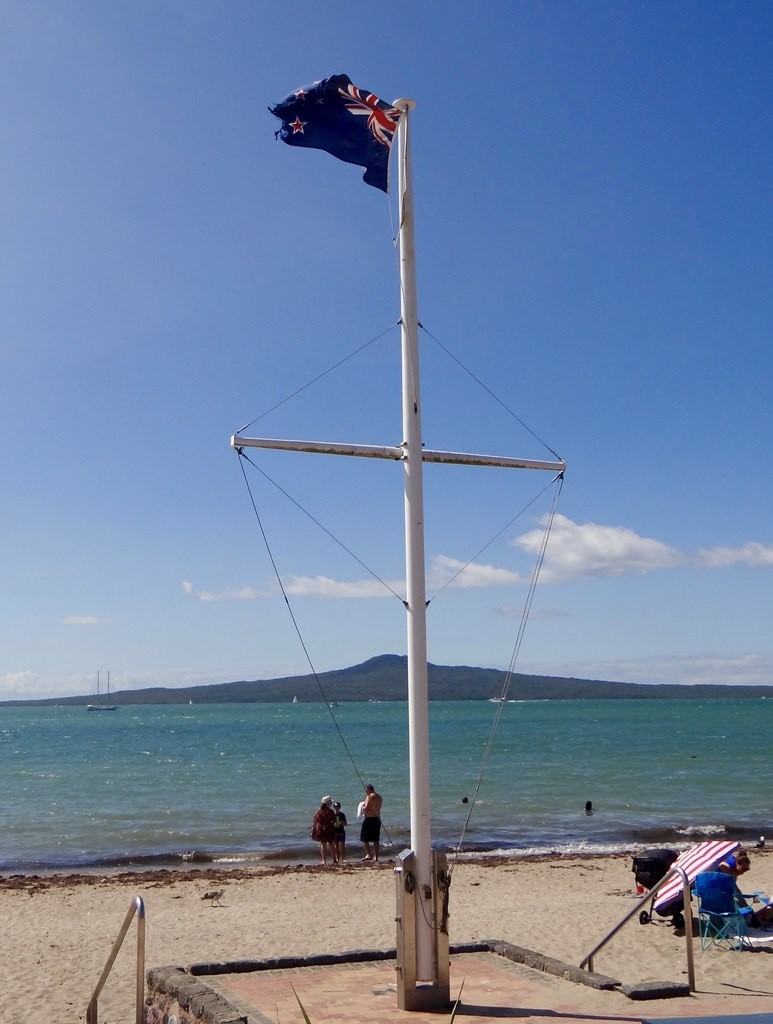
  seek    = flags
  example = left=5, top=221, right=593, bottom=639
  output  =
left=267, top=74, right=402, bottom=194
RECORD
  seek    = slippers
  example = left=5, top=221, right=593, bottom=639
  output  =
left=361, top=857, right=372, bottom=861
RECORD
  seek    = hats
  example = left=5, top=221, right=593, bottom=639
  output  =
left=321, top=797, right=332, bottom=806
left=334, top=802, right=341, bottom=808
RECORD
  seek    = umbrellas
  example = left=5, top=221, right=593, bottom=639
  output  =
left=655, top=841, right=742, bottom=917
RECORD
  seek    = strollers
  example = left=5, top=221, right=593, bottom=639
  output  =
left=631, top=848, right=694, bottom=930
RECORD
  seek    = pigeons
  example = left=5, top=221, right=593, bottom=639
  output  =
left=200, top=888, right=226, bottom=907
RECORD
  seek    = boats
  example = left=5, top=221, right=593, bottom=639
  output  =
left=329, top=702, right=343, bottom=707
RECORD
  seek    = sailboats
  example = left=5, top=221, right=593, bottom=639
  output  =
left=86, top=670, right=118, bottom=711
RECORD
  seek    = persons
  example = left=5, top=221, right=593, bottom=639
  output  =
left=313, top=795, right=339, bottom=863
left=331, top=801, right=347, bottom=862
left=360, top=784, right=382, bottom=862
left=711, top=850, right=759, bottom=939
left=462, top=797, right=469, bottom=804
left=584, top=801, right=594, bottom=811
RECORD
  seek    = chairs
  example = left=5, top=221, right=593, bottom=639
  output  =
left=694, top=872, right=754, bottom=952
